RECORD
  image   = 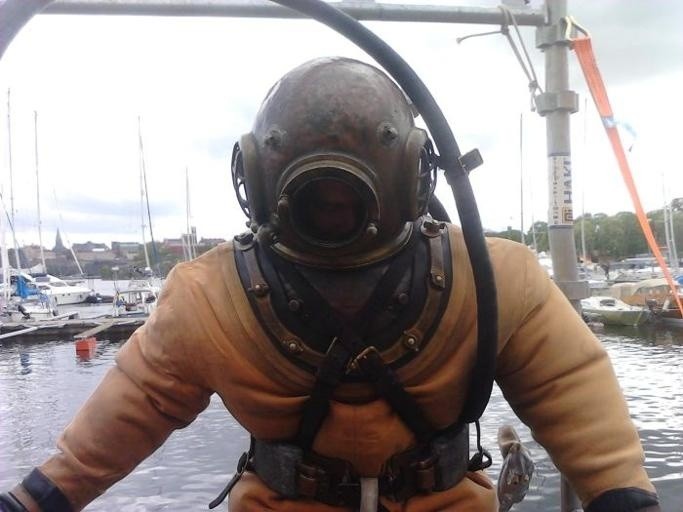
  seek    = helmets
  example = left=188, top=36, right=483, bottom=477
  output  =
left=229, top=55, right=439, bottom=274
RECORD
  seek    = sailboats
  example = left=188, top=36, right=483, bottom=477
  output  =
left=520, top=98, right=683, bottom=329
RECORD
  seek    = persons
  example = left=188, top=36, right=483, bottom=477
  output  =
left=0, top=56, right=658, bottom=512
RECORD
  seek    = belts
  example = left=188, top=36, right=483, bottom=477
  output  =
left=250, top=421, right=469, bottom=507
